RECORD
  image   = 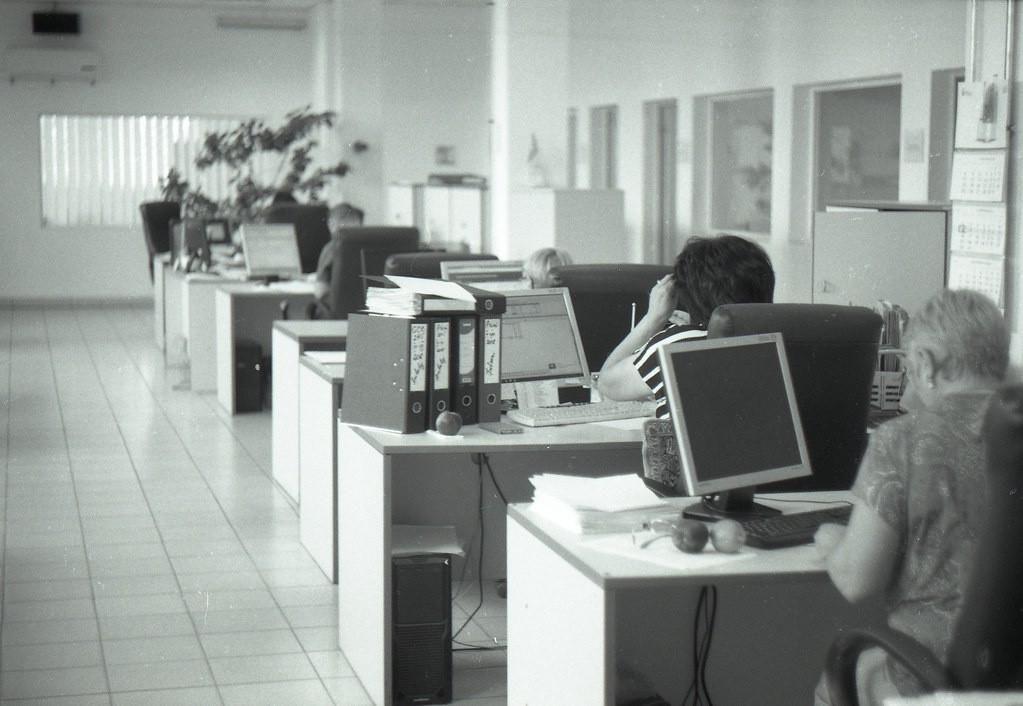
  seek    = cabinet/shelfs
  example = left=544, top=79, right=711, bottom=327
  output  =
left=508, top=184, right=626, bottom=261
left=806, top=198, right=955, bottom=310
left=385, top=183, right=422, bottom=249
left=422, top=185, right=487, bottom=253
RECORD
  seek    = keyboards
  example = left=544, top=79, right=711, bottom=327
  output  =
left=740, top=504, right=855, bottom=548
left=507, top=401, right=654, bottom=427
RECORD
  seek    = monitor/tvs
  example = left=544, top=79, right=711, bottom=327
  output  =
left=167, top=217, right=214, bottom=274
left=498, top=286, right=591, bottom=409
left=441, top=260, right=529, bottom=291
left=657, top=331, right=811, bottom=524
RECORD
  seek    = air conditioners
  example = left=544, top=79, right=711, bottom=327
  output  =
left=5, top=43, right=101, bottom=85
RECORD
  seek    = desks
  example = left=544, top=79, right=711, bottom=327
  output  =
left=184, top=269, right=248, bottom=390
left=147, top=247, right=238, bottom=355
left=269, top=315, right=349, bottom=504
left=213, top=282, right=317, bottom=411
left=335, top=407, right=658, bottom=706
left=506, top=486, right=871, bottom=706
left=292, top=350, right=346, bottom=584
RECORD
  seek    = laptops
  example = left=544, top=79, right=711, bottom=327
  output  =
left=239, top=222, right=309, bottom=282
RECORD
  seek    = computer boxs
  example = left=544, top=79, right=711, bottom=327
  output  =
left=390, top=550, right=453, bottom=706
left=237, top=341, right=265, bottom=414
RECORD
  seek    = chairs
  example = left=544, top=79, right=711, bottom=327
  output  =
left=139, top=197, right=184, bottom=289
left=255, top=199, right=332, bottom=271
left=279, top=212, right=422, bottom=318
left=554, top=259, right=672, bottom=368
left=642, top=296, right=893, bottom=496
left=828, top=382, right=1023, bottom=706
left=386, top=250, right=498, bottom=281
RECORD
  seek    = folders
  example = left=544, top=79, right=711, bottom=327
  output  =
left=356, top=274, right=506, bottom=317
left=879, top=299, right=910, bottom=372
left=340, top=312, right=427, bottom=435
left=428, top=317, right=454, bottom=435
left=453, top=317, right=475, bottom=427
left=479, top=316, right=502, bottom=422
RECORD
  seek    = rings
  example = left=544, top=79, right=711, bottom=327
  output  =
left=657, top=280, right=660, bottom=284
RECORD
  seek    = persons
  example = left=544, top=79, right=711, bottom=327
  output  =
left=598, top=235, right=776, bottom=420
left=312, top=204, right=365, bottom=318
left=816, top=288, right=1023, bottom=706
left=523, top=248, right=572, bottom=288
